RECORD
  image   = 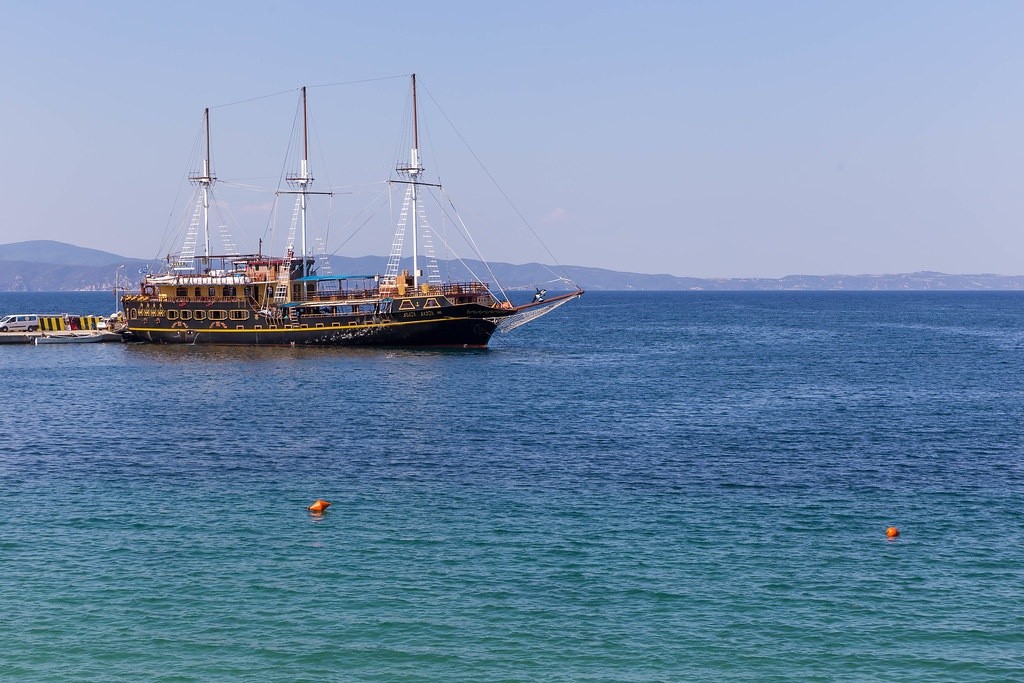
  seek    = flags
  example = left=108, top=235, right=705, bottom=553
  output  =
left=139, top=267, right=147, bottom=273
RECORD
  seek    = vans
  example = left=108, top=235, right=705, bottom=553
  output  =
left=0, top=314, right=40, bottom=332
left=97, top=318, right=111, bottom=330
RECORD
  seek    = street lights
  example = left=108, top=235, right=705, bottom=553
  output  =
left=114, top=265, right=125, bottom=313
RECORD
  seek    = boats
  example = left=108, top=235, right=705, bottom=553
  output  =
left=106, top=72, right=588, bottom=352
left=23, top=330, right=122, bottom=346
